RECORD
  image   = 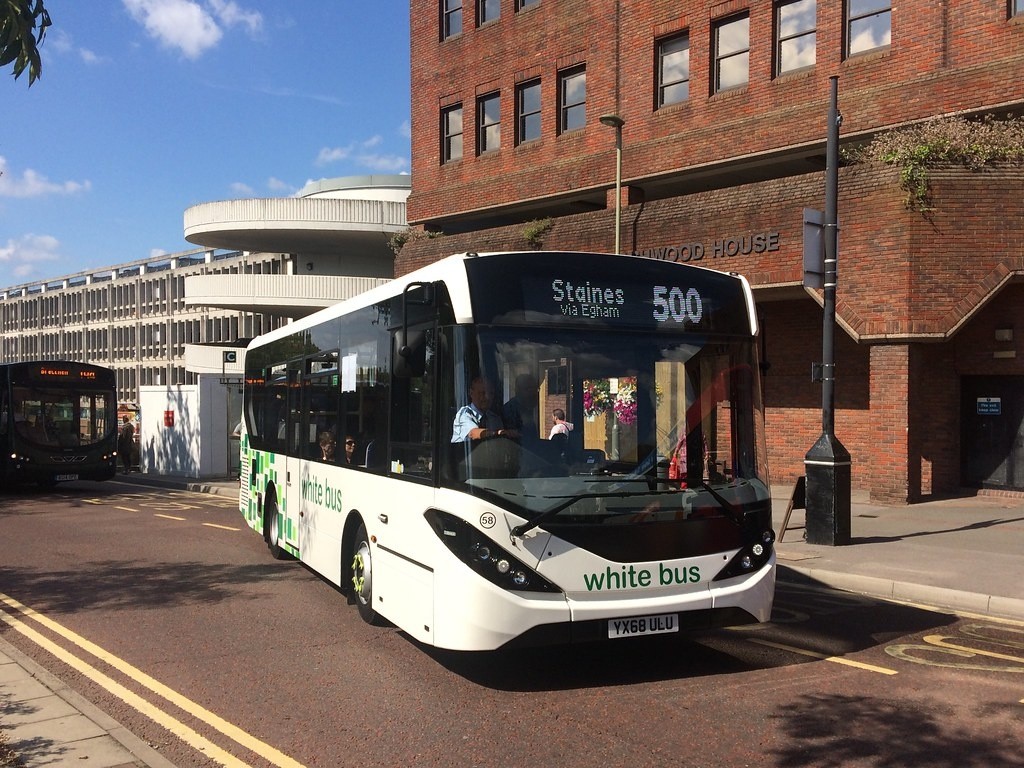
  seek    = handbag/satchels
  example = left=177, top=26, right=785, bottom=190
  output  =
left=669, top=458, right=680, bottom=479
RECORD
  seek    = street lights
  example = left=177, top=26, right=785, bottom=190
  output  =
left=600, top=114, right=625, bottom=254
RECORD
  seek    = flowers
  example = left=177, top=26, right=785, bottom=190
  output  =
left=583, top=379, right=613, bottom=421
left=614, top=377, right=663, bottom=423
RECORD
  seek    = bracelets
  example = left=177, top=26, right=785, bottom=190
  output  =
left=496, top=429, right=500, bottom=436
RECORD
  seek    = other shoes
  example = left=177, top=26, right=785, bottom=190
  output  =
left=122, top=470, right=129, bottom=475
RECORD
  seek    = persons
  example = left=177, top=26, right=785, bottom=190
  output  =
left=232, top=422, right=241, bottom=437
left=319, top=432, right=336, bottom=461
left=0, top=399, right=25, bottom=424
left=345, top=434, right=355, bottom=464
left=502, top=374, right=538, bottom=437
left=450, top=375, right=523, bottom=443
left=548, top=409, right=574, bottom=440
left=120, top=415, right=135, bottom=474
left=669, top=428, right=709, bottom=490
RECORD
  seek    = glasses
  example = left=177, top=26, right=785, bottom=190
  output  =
left=346, top=441, right=357, bottom=445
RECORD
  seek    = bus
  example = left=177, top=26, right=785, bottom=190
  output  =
left=237, top=248, right=776, bottom=656
left=0, top=360, right=118, bottom=488
